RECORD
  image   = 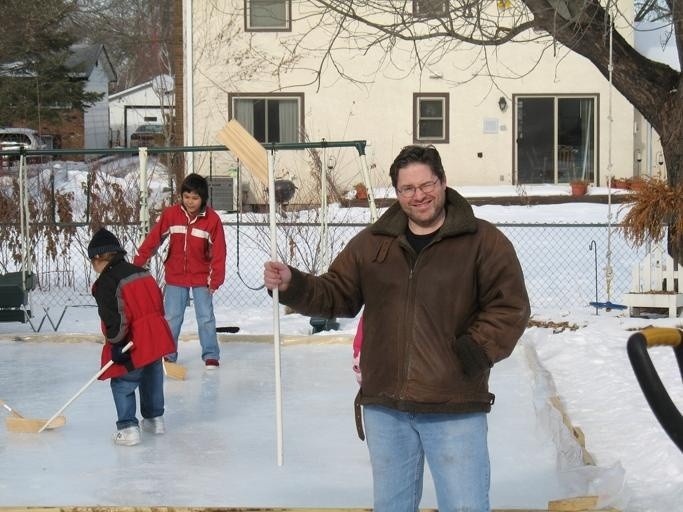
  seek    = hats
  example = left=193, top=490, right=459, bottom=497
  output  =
left=85, top=225, right=124, bottom=259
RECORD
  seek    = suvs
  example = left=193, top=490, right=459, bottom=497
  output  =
left=0, top=128, right=47, bottom=167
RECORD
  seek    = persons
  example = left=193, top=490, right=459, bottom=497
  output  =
left=88, top=227, right=177, bottom=446
left=264, top=143, right=532, bottom=512
left=131, top=172, right=227, bottom=379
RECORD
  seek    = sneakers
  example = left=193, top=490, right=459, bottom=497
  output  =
left=205, top=358, right=221, bottom=369
left=139, top=414, right=167, bottom=435
left=112, top=424, right=144, bottom=447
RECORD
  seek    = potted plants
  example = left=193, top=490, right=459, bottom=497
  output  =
left=606, top=175, right=649, bottom=191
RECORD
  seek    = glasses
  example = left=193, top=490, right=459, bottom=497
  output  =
left=397, top=177, right=443, bottom=198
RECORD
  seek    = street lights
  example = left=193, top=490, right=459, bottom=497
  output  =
left=30, top=70, right=41, bottom=142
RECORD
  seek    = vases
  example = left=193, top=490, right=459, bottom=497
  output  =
left=568, top=182, right=589, bottom=196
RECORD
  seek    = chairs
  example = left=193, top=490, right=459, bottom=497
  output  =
left=525, top=142, right=579, bottom=183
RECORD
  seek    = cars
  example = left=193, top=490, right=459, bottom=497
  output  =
left=131, top=123, right=171, bottom=150
